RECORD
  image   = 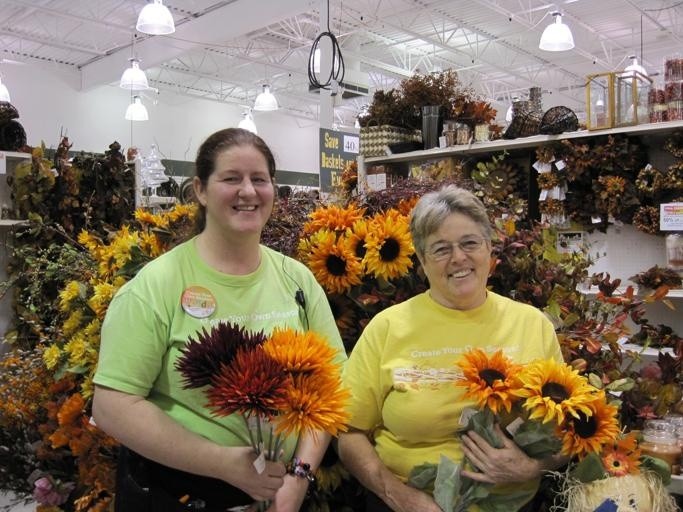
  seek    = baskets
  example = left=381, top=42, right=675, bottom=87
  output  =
left=359, top=118, right=424, bottom=157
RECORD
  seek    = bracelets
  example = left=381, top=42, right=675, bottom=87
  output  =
left=285, top=456, right=315, bottom=482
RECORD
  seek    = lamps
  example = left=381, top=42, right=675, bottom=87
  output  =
left=237, top=61, right=280, bottom=135
left=538, top=1, right=576, bottom=52
left=121, top=0, right=177, bottom=122
left=622, top=54, right=650, bottom=87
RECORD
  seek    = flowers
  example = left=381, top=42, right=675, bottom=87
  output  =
left=33, top=183, right=678, bottom=510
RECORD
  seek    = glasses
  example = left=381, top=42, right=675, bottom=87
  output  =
left=424, top=234, right=489, bottom=261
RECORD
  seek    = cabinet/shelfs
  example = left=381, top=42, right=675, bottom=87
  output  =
left=355, top=115, right=683, bottom=297
left=0, top=151, right=43, bottom=226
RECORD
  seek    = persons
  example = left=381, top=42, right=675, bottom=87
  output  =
left=92, top=128, right=348, bottom=512
left=331, top=184, right=571, bottom=512
